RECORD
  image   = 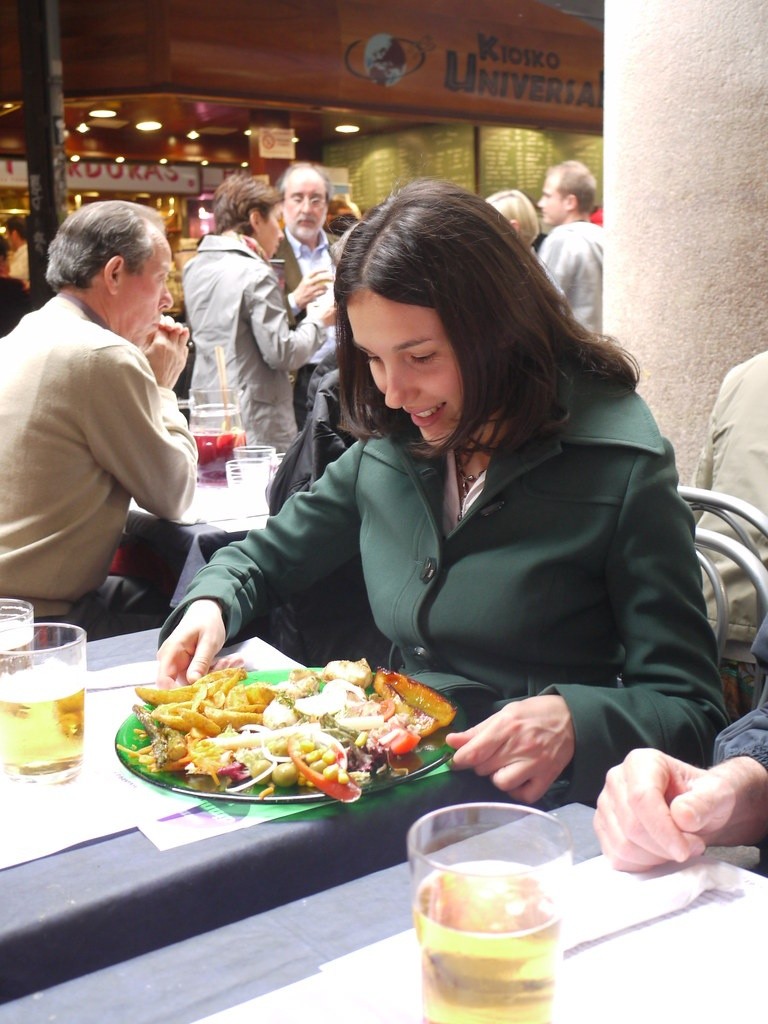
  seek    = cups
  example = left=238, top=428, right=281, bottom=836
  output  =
left=0, top=622, right=87, bottom=786
left=225, top=446, right=286, bottom=507
left=313, top=263, right=332, bottom=289
left=406, top=801, right=573, bottom=1023
left=0, top=598, right=34, bottom=673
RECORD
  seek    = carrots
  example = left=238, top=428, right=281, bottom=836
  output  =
left=113, top=725, right=231, bottom=788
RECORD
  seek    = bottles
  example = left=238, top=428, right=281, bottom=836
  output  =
left=189, top=386, right=245, bottom=486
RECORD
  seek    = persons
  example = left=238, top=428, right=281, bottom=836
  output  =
left=179, top=160, right=605, bottom=452
left=0, top=215, right=30, bottom=340
left=1, top=200, right=198, bottom=646
left=688, top=351, right=768, bottom=661
left=157, top=180, right=731, bottom=810
left=593, top=609, right=768, bottom=877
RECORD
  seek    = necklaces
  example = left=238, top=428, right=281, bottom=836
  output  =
left=453, top=448, right=485, bottom=520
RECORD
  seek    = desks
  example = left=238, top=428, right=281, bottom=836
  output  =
left=1, top=618, right=499, bottom=1001
left=0, top=800, right=768, bottom=1024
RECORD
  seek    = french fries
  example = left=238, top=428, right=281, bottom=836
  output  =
left=136, top=667, right=278, bottom=737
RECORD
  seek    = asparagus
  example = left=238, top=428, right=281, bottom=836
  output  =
left=132, top=705, right=188, bottom=766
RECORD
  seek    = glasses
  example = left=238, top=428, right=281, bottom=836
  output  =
left=283, top=196, right=327, bottom=209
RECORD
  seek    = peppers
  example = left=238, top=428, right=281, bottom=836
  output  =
left=287, top=664, right=458, bottom=803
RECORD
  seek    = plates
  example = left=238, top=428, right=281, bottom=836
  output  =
left=115, top=668, right=456, bottom=805
